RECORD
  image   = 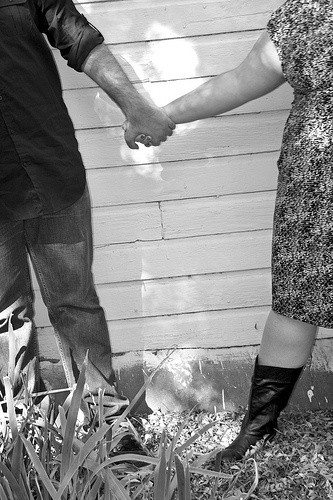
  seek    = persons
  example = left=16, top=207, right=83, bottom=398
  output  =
left=0, top=0, right=175, bottom=469
left=121, top=0, right=332, bottom=473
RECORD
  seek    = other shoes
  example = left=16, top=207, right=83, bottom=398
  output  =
left=105, top=423, right=147, bottom=466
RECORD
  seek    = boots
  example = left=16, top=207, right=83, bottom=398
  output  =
left=205, top=356, right=305, bottom=469
